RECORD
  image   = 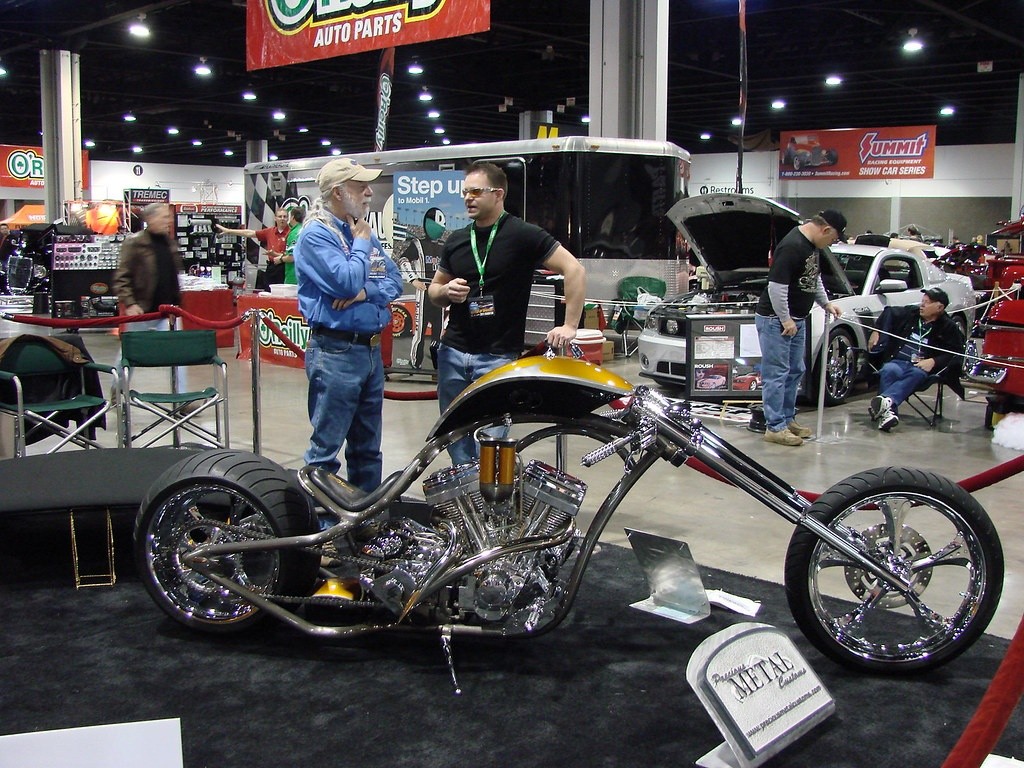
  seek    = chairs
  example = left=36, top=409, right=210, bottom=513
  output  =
left=616, top=275, right=669, bottom=360
left=902, top=364, right=952, bottom=428
left=115, top=329, right=235, bottom=449
left=1, top=331, right=123, bottom=460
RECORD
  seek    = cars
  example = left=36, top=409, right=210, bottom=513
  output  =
left=639, top=192, right=976, bottom=404
left=782, top=134, right=839, bottom=171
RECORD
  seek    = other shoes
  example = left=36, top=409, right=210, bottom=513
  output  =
left=110, top=388, right=117, bottom=408
left=183, top=402, right=200, bottom=415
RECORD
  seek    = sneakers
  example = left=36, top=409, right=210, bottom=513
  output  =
left=765, top=426, right=803, bottom=446
left=320, top=541, right=342, bottom=568
left=879, top=411, right=898, bottom=431
left=787, top=420, right=812, bottom=439
left=868, top=395, right=888, bottom=420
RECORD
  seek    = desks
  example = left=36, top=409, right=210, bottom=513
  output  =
left=229, top=293, right=396, bottom=384
left=174, top=284, right=239, bottom=348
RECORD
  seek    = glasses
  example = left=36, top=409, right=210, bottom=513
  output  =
left=462, top=187, right=499, bottom=197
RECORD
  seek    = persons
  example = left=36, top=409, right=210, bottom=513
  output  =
left=866, top=286, right=966, bottom=433
left=675, top=233, right=704, bottom=299
left=215, top=208, right=291, bottom=295
left=864, top=223, right=1017, bottom=256
left=755, top=206, right=852, bottom=448
left=292, top=157, right=405, bottom=538
left=110, top=201, right=206, bottom=417
left=423, top=160, right=588, bottom=473
left=0, top=222, right=19, bottom=276
left=273, top=204, right=305, bottom=286
left=114, top=225, right=129, bottom=243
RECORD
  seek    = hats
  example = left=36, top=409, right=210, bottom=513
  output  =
left=920, top=287, right=949, bottom=308
left=315, top=158, right=385, bottom=192
left=818, top=208, right=848, bottom=244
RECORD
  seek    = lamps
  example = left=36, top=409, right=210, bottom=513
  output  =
left=2, top=11, right=456, bottom=158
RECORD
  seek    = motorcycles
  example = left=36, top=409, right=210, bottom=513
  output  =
left=128, top=354, right=1006, bottom=695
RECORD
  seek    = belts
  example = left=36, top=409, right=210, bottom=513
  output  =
left=317, top=327, right=382, bottom=346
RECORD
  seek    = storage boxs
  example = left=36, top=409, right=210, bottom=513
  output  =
left=583, top=301, right=616, bottom=362
